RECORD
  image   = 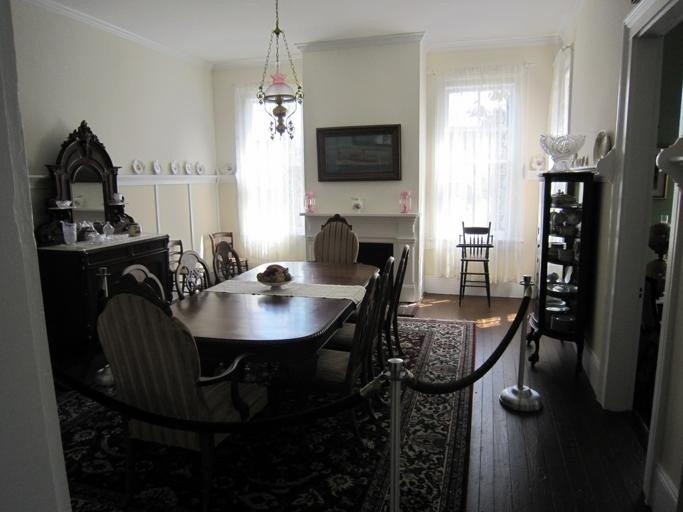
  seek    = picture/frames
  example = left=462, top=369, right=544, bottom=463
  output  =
left=314, top=124, right=401, bottom=182
left=652, top=145, right=668, bottom=200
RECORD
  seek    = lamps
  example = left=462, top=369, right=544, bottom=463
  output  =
left=255, top=3, right=307, bottom=146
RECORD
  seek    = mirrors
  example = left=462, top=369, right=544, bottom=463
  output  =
left=68, top=180, right=106, bottom=232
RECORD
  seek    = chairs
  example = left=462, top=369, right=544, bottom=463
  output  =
left=86, top=285, right=271, bottom=512
left=312, top=215, right=363, bottom=262
left=298, top=245, right=410, bottom=449
left=459, top=224, right=491, bottom=308
left=168, top=229, right=252, bottom=299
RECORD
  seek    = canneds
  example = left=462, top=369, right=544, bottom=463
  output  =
left=127, top=223, right=141, bottom=236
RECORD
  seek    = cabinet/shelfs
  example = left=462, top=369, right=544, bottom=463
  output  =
left=41, top=238, right=168, bottom=391
left=526, top=179, right=599, bottom=376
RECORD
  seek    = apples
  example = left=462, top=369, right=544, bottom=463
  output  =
left=261, top=265, right=290, bottom=283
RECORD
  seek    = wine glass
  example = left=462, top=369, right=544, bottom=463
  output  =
left=114, top=193, right=122, bottom=205
left=257, top=276, right=296, bottom=291
left=539, top=132, right=585, bottom=173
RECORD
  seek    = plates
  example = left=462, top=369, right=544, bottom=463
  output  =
left=132, top=158, right=205, bottom=175
left=592, top=129, right=611, bottom=163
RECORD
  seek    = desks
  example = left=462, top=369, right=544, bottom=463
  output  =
left=168, top=259, right=381, bottom=413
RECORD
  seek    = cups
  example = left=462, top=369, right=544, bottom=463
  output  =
left=661, top=214, right=668, bottom=224
left=398, top=191, right=415, bottom=213
left=351, top=196, right=361, bottom=215
left=60, top=221, right=77, bottom=247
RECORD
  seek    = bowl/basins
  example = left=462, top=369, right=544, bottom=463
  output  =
left=55, top=200, right=72, bottom=208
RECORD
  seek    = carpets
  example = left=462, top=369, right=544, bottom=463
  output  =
left=45, top=317, right=476, bottom=512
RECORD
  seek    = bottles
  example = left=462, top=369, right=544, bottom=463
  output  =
left=85, top=221, right=114, bottom=244
left=303, top=191, right=316, bottom=214
